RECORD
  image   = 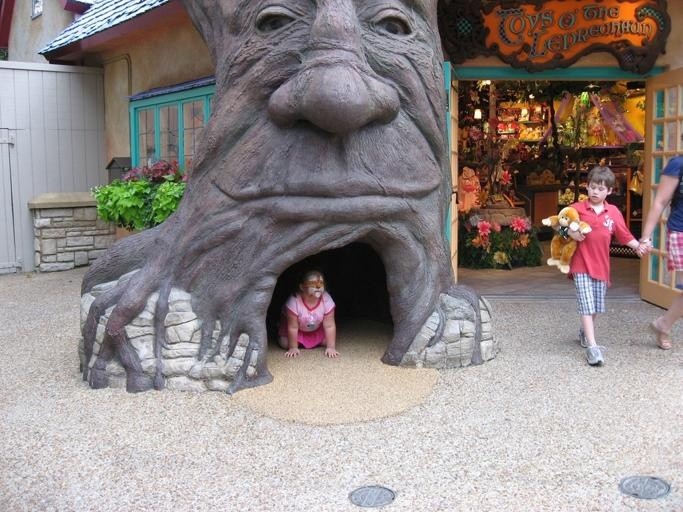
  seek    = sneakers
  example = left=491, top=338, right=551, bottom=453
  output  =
left=583, top=345, right=604, bottom=365
left=579, top=328, right=589, bottom=348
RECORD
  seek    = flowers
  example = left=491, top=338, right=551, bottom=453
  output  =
left=462, top=214, right=543, bottom=271
left=491, top=170, right=512, bottom=201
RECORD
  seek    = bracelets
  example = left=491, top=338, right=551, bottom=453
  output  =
left=638, top=237, right=652, bottom=245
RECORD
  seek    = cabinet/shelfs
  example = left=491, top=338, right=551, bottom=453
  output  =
left=557, top=148, right=645, bottom=247
left=497, top=102, right=548, bottom=142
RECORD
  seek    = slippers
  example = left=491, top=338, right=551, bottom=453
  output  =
left=650, top=322, right=673, bottom=350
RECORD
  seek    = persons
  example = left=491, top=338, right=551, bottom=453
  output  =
left=632, top=130, right=683, bottom=351
left=564, top=165, right=654, bottom=368
left=278, top=268, right=339, bottom=359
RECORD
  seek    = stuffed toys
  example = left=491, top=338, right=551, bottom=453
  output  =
left=541, top=205, right=588, bottom=275
